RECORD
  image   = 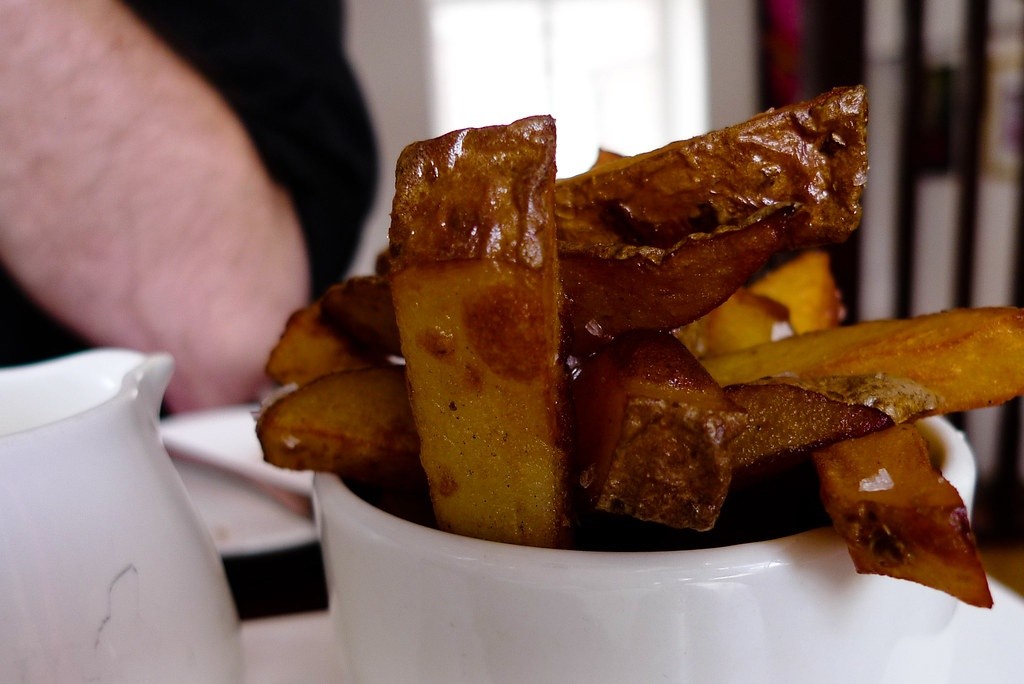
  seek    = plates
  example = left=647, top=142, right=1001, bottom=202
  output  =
left=159, top=406, right=316, bottom=556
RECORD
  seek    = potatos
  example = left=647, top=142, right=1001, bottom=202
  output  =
left=255, top=85, right=1024, bottom=609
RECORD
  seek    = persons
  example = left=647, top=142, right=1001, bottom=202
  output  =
left=0, top=0, right=379, bottom=420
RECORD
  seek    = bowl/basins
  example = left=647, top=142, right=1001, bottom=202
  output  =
left=310, top=414, right=974, bottom=683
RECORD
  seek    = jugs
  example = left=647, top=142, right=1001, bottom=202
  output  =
left=0, top=346, right=247, bottom=681
